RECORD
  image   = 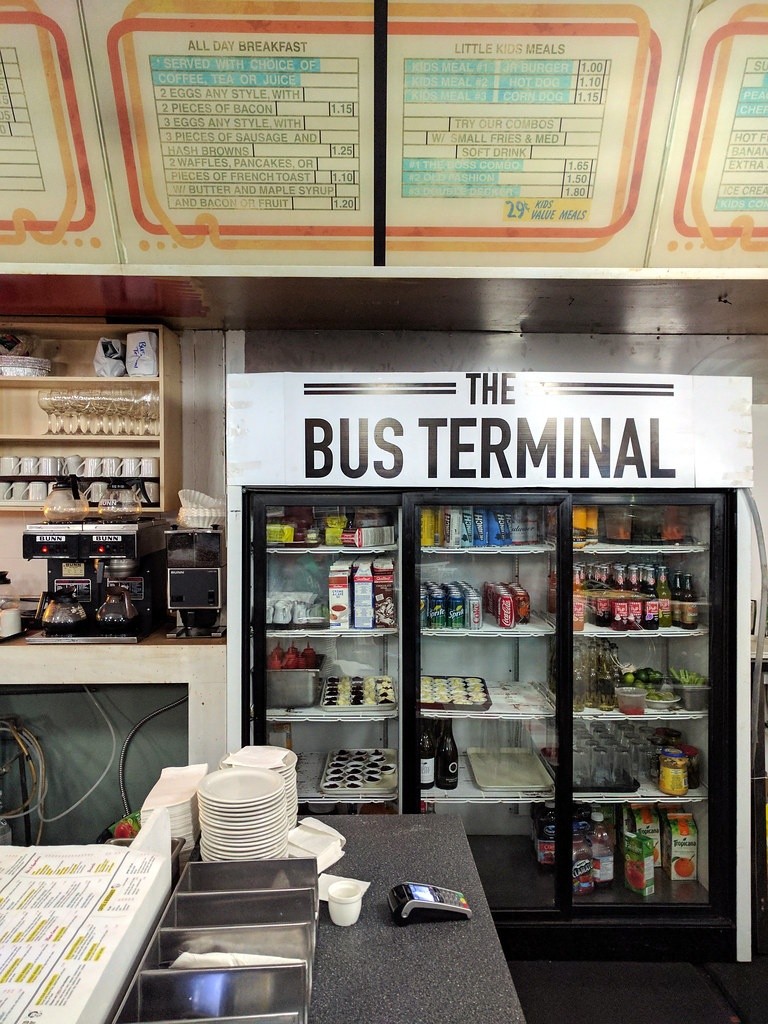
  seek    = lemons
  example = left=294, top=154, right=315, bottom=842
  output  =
left=623, top=667, right=665, bottom=690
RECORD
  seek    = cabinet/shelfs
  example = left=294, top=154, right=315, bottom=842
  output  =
left=0, top=325, right=182, bottom=515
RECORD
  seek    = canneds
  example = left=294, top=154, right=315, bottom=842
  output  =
left=647, top=727, right=701, bottom=797
left=418, top=581, right=532, bottom=631
left=419, top=505, right=540, bottom=547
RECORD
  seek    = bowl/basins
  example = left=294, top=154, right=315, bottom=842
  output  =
left=645, top=695, right=680, bottom=709
left=615, top=686, right=648, bottom=714
left=421, top=676, right=487, bottom=705
left=673, top=684, right=710, bottom=710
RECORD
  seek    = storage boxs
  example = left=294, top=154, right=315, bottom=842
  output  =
left=328, top=526, right=396, bottom=630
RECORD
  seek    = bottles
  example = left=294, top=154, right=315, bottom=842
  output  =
left=529, top=803, right=617, bottom=895
left=426, top=803, right=436, bottom=814
left=573, top=636, right=621, bottom=712
left=266, top=642, right=318, bottom=669
left=421, top=718, right=459, bottom=789
left=0, top=570, right=21, bottom=639
left=548, top=571, right=557, bottom=612
left=572, top=561, right=700, bottom=633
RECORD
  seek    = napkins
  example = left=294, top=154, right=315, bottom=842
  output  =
left=223, top=745, right=290, bottom=769
left=140, top=761, right=210, bottom=852
left=286, top=816, right=372, bottom=903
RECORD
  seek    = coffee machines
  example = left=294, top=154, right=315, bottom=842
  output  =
left=21, top=519, right=166, bottom=644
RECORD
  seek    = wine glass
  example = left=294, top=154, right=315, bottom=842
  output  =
left=38, top=383, right=160, bottom=437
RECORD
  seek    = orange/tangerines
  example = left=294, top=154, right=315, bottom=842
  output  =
left=653, top=847, right=693, bottom=877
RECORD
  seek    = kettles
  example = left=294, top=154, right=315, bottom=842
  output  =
left=98, top=479, right=152, bottom=519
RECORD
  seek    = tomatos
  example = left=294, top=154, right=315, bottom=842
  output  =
left=114, top=823, right=138, bottom=841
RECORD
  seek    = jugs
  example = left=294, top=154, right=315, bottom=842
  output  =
left=43, top=473, right=89, bottom=521
left=35, top=585, right=87, bottom=632
left=95, top=586, right=138, bottom=633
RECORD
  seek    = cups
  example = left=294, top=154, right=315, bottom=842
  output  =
left=265, top=599, right=324, bottom=624
left=328, top=881, right=362, bottom=926
left=0, top=455, right=159, bottom=504
left=545, top=719, right=655, bottom=788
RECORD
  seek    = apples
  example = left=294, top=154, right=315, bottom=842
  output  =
left=626, top=859, right=646, bottom=889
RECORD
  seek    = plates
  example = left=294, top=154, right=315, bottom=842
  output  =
left=322, top=751, right=397, bottom=790
left=178, top=489, right=226, bottom=529
left=322, top=676, right=395, bottom=707
left=195, top=746, right=299, bottom=862
